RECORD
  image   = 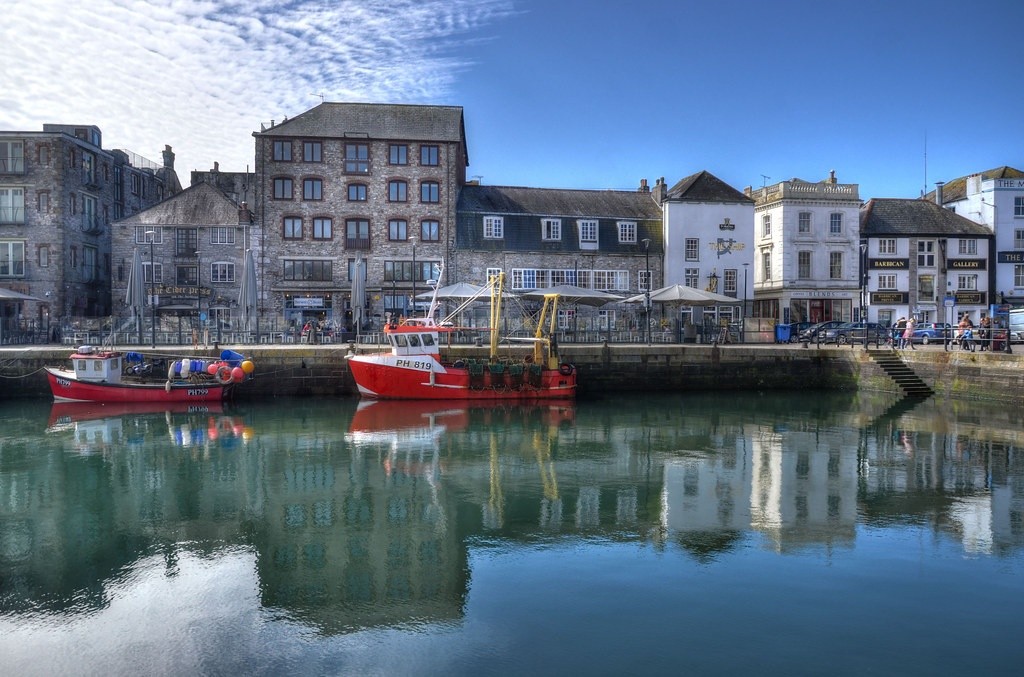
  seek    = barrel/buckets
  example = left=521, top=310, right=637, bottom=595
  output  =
left=220, top=350, right=245, bottom=367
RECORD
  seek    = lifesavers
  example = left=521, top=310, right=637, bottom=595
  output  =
left=215, top=365, right=235, bottom=385
left=125, top=366, right=134, bottom=376
left=559, top=362, right=573, bottom=376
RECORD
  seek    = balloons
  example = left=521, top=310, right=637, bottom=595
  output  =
left=207, top=361, right=255, bottom=383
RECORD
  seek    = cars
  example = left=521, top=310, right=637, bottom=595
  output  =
left=774, top=321, right=819, bottom=344
left=952, top=324, right=983, bottom=345
left=819, top=322, right=889, bottom=346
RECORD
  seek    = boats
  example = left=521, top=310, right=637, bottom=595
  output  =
left=42, top=344, right=254, bottom=404
left=45, top=398, right=252, bottom=461
left=343, top=272, right=579, bottom=400
left=341, top=390, right=577, bottom=530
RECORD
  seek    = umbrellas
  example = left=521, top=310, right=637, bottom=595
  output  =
left=0, top=287, right=43, bottom=303
left=617, top=283, right=743, bottom=343
left=237, top=248, right=258, bottom=337
left=414, top=282, right=519, bottom=343
left=522, top=285, right=628, bottom=342
left=349, top=251, right=368, bottom=344
left=125, top=247, right=146, bottom=339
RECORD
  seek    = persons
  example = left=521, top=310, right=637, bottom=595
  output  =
left=896, top=317, right=907, bottom=349
left=957, top=313, right=991, bottom=352
left=302, top=322, right=347, bottom=343
left=384, top=319, right=436, bottom=346
left=902, top=317, right=917, bottom=350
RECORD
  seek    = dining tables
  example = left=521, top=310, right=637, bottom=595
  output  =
left=309, top=329, right=323, bottom=344
left=515, top=331, right=529, bottom=344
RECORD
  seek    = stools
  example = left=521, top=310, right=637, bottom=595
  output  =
left=323, top=335, right=330, bottom=343
left=301, top=335, right=307, bottom=344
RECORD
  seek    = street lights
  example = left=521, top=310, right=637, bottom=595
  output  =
left=194, top=250, right=202, bottom=343
left=407, top=235, right=420, bottom=320
left=642, top=237, right=653, bottom=346
left=859, top=242, right=869, bottom=347
left=145, top=230, right=160, bottom=349
left=742, top=262, right=751, bottom=318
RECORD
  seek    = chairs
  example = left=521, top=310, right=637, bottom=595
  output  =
left=62, top=330, right=294, bottom=345
left=555, top=330, right=673, bottom=343
left=354, top=331, right=388, bottom=343
left=437, top=331, right=490, bottom=344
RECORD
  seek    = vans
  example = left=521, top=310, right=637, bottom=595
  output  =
left=911, top=322, right=951, bottom=345
left=1008, top=308, right=1024, bottom=343
left=800, top=321, right=847, bottom=344
